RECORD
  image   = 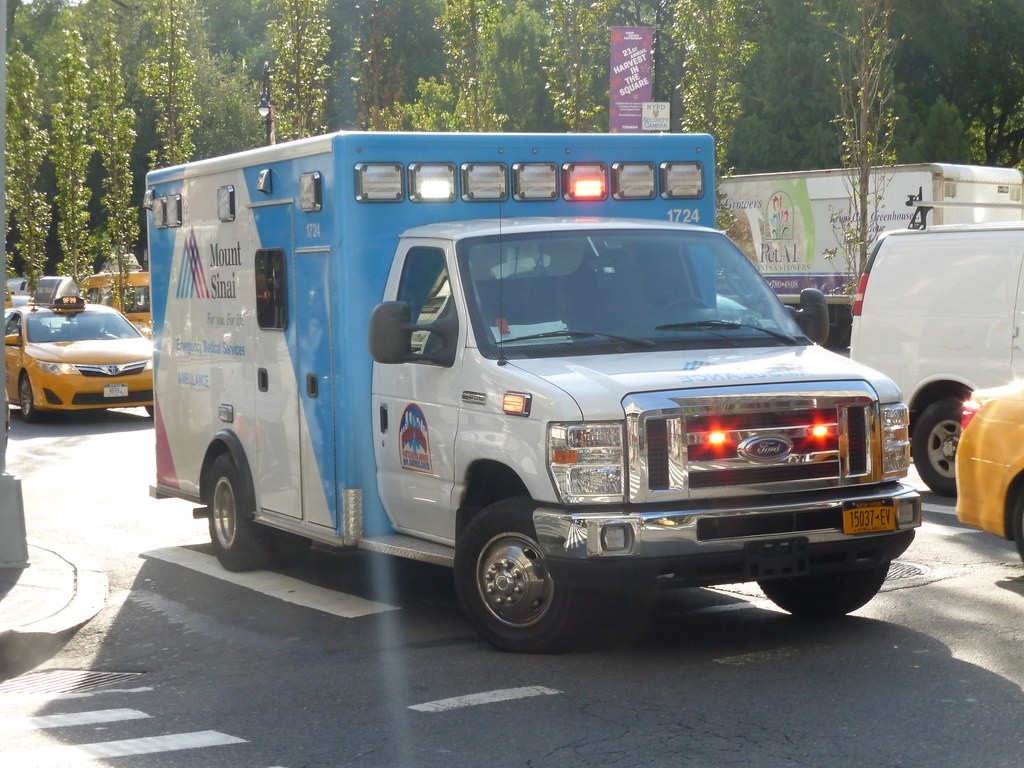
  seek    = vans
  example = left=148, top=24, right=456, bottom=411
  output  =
left=850, top=187, right=1024, bottom=499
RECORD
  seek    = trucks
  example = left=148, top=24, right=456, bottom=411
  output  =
left=716, top=162, right=1024, bottom=349
left=144, top=130, right=922, bottom=653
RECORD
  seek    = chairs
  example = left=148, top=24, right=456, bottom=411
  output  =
left=59, top=322, right=80, bottom=340
left=28, top=320, right=56, bottom=342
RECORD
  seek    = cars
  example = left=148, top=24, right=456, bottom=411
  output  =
left=80, top=253, right=150, bottom=340
left=954, top=384, right=1024, bottom=563
left=0, top=276, right=153, bottom=422
